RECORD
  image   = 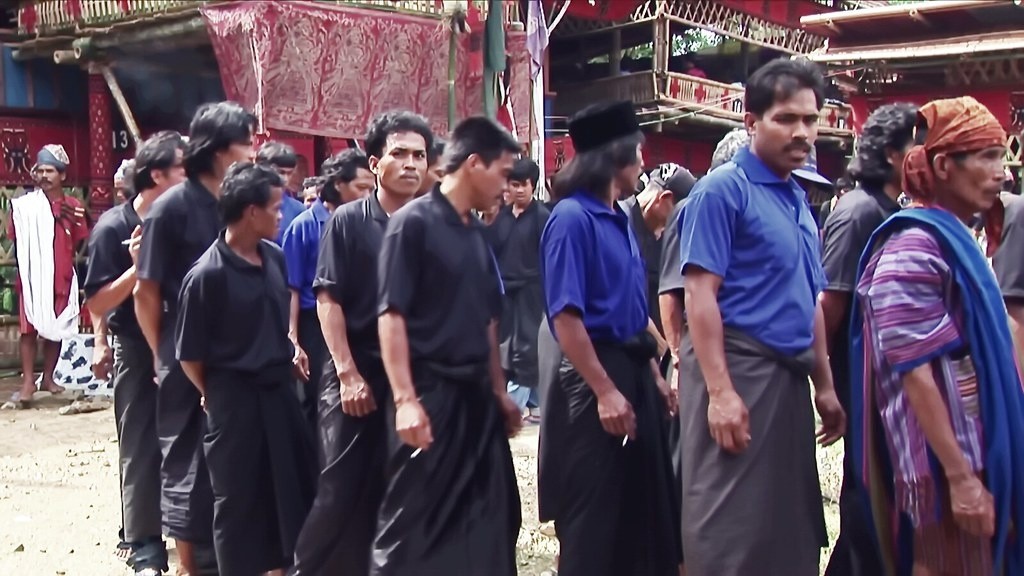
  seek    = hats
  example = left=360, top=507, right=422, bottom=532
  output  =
left=791, top=144, right=833, bottom=189
left=650, top=162, right=696, bottom=201
left=565, top=99, right=642, bottom=154
left=37, top=143, right=70, bottom=169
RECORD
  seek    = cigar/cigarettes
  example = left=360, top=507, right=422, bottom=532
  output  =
left=622, top=434, right=629, bottom=445
left=410, top=447, right=422, bottom=458
left=122, top=239, right=134, bottom=244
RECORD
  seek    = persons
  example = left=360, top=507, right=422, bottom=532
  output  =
left=85, top=53, right=1024, bottom=576
left=7, top=143, right=92, bottom=402
